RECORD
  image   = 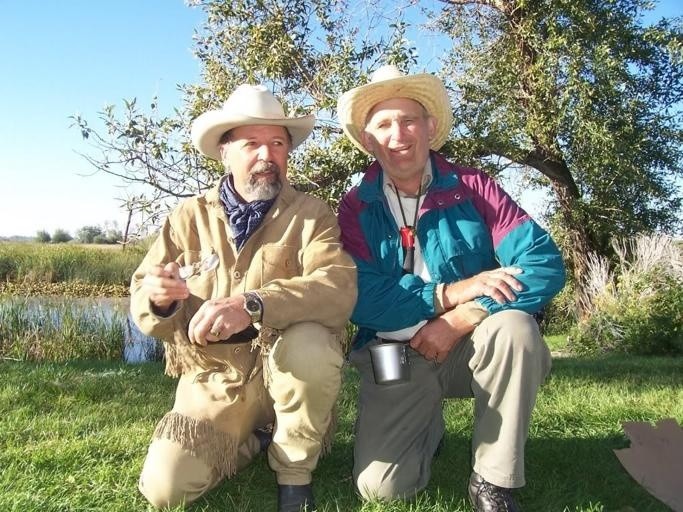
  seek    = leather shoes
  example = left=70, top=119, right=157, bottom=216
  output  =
left=253, top=427, right=275, bottom=455
left=275, top=479, right=313, bottom=512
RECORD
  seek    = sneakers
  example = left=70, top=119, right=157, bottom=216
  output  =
left=467, top=470, right=521, bottom=512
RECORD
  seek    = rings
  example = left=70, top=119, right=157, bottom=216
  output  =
left=208, top=329, right=220, bottom=340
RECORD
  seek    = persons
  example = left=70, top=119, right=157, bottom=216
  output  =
left=119, top=82, right=358, bottom=512
left=331, top=61, right=568, bottom=511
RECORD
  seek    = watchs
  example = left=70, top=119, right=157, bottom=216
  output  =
left=238, top=288, right=263, bottom=326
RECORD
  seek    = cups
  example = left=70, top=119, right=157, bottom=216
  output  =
left=366, top=342, right=414, bottom=387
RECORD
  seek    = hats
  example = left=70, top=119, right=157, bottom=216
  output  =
left=335, top=63, right=453, bottom=159
left=189, top=82, right=316, bottom=160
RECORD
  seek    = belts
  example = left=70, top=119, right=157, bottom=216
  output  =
left=373, top=334, right=410, bottom=346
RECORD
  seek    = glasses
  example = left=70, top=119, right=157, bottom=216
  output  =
left=174, top=250, right=220, bottom=282
